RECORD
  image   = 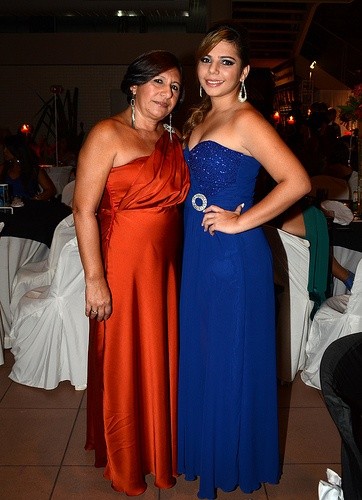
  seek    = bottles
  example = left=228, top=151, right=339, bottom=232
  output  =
left=273, top=88, right=295, bottom=111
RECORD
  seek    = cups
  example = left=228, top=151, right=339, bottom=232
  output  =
left=0, top=184, right=9, bottom=207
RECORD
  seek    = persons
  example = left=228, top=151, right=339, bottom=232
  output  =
left=72, top=50, right=190, bottom=496
left=176, top=28, right=312, bottom=500
left=268, top=101, right=362, bottom=319
left=318, top=332, right=362, bottom=500
left=0, top=134, right=80, bottom=248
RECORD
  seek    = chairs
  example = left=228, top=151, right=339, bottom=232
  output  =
left=261, top=224, right=362, bottom=390
left=0, top=171, right=95, bottom=390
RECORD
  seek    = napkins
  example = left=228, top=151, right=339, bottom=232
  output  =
left=321, top=199, right=353, bottom=225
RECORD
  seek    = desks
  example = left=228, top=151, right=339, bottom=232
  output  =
left=0, top=200, right=71, bottom=365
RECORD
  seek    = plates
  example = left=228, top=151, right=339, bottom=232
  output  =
left=11, top=204, right=24, bottom=207
left=352, top=219, right=362, bottom=222
